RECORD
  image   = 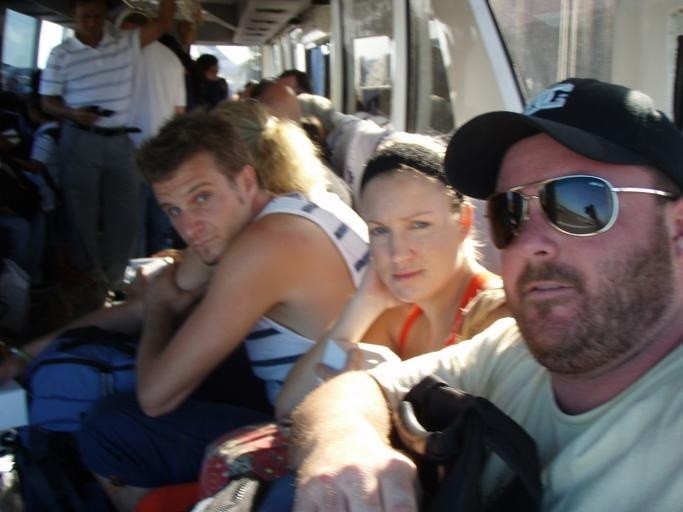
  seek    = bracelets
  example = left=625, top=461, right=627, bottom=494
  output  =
left=9, top=347, right=32, bottom=362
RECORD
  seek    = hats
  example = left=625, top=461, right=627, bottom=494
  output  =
left=433, top=80, right=681, bottom=191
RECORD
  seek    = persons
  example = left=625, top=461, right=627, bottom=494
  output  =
left=1, top=112, right=371, bottom=510
left=38, top=2, right=177, bottom=293
left=0, top=71, right=78, bottom=322
left=238, top=69, right=327, bottom=162
left=192, top=75, right=681, bottom=510
left=128, top=99, right=322, bottom=302
left=262, top=126, right=510, bottom=511
left=160, top=22, right=228, bottom=113
left=116, top=11, right=187, bottom=258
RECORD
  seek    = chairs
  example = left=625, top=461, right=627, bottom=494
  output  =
left=296, top=91, right=507, bottom=276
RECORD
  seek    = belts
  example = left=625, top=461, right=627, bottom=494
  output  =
left=55, top=117, right=144, bottom=140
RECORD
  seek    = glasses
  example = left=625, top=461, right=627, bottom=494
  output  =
left=477, top=168, right=674, bottom=248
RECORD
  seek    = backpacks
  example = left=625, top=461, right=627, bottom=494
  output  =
left=26, top=327, right=150, bottom=433
left=190, top=371, right=544, bottom=511
